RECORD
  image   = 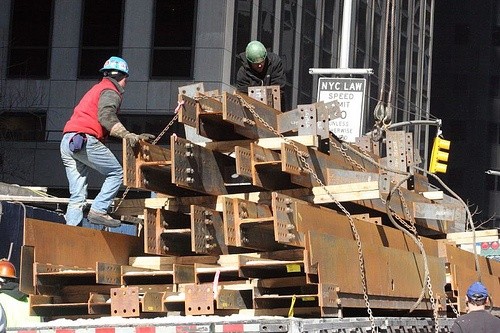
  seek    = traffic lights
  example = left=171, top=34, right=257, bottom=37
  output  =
left=429, top=137, right=450, bottom=174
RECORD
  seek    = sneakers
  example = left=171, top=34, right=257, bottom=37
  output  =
left=86, top=208, right=122, bottom=229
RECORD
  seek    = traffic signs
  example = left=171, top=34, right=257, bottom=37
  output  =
left=317, top=77, right=367, bottom=141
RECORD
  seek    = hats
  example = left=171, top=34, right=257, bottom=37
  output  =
left=466, top=282, right=488, bottom=302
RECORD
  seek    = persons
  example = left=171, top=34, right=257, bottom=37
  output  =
left=450, top=281, right=500, bottom=333
left=59, top=55, right=156, bottom=228
left=0, top=258, right=45, bottom=333
left=237, top=40, right=288, bottom=113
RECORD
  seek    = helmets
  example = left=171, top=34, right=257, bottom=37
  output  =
left=0, top=261, right=19, bottom=280
left=245, top=40, right=268, bottom=64
left=98, top=56, right=130, bottom=76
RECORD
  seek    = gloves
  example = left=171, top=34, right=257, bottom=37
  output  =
left=134, top=134, right=156, bottom=142
left=110, top=122, right=144, bottom=148
